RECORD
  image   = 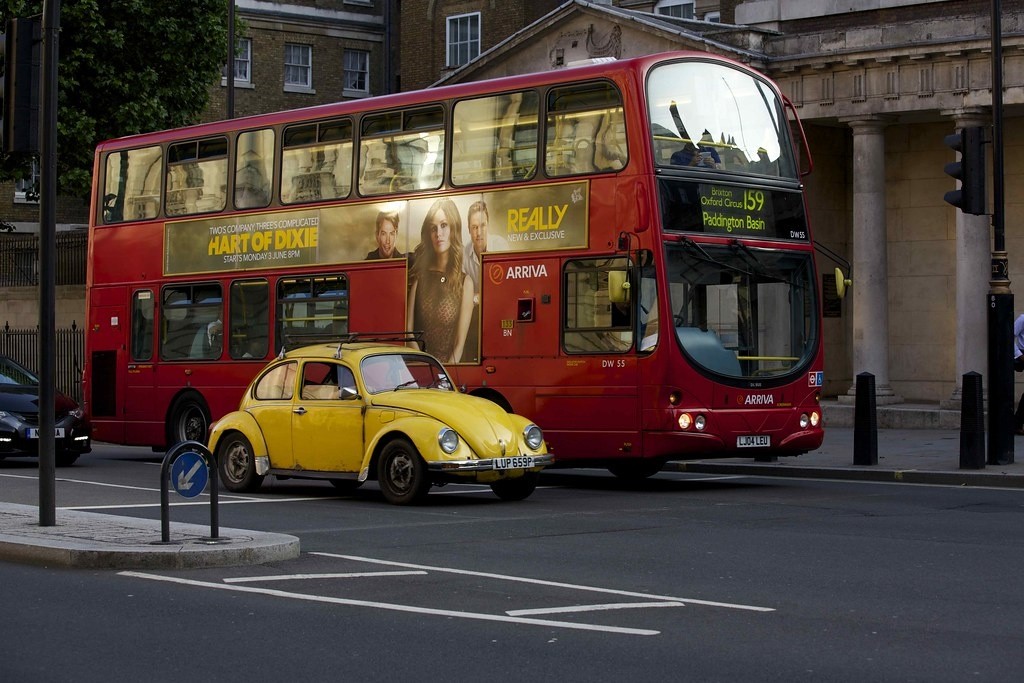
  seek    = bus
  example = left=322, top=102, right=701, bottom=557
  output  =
left=80, top=52, right=852, bottom=480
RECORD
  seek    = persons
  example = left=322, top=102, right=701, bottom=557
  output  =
left=1014, top=311, right=1024, bottom=436
left=206, top=305, right=244, bottom=357
left=669, top=127, right=722, bottom=170
left=463, top=202, right=510, bottom=349
left=407, top=195, right=473, bottom=364
left=365, top=209, right=414, bottom=266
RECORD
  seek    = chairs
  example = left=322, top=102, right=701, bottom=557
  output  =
left=135, top=319, right=324, bottom=355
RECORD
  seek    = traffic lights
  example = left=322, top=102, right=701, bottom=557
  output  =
left=945, top=126, right=985, bottom=215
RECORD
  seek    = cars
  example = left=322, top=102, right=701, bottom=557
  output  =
left=208, top=330, right=555, bottom=504
left=0, top=350, right=91, bottom=468
